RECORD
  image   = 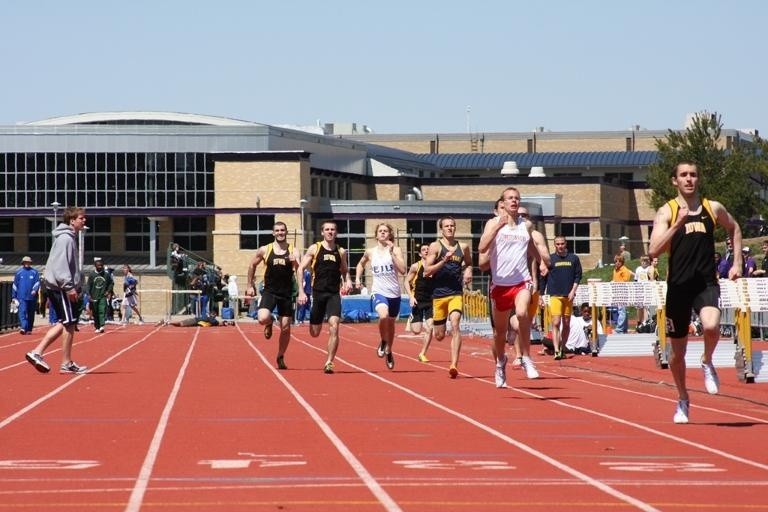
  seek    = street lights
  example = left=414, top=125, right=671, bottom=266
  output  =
left=300, top=198, right=306, bottom=256
left=619, top=236, right=630, bottom=248
left=77, top=224, right=89, bottom=272
left=49, top=201, right=61, bottom=228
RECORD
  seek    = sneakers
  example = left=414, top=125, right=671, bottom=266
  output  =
left=26, top=351, right=50, bottom=373
left=449, top=364, right=458, bottom=379
left=377, top=340, right=394, bottom=369
left=60, top=361, right=87, bottom=374
left=324, top=361, right=335, bottom=373
left=49, top=320, right=129, bottom=333
left=264, top=315, right=274, bottom=339
left=496, top=353, right=507, bottom=388
left=512, top=356, right=539, bottom=379
left=138, top=316, right=144, bottom=326
left=701, top=354, right=720, bottom=395
left=673, top=399, right=688, bottom=423
left=20, top=328, right=32, bottom=335
left=554, top=345, right=566, bottom=360
left=419, top=353, right=430, bottom=362
left=276, top=354, right=287, bottom=369
left=40, top=314, right=47, bottom=319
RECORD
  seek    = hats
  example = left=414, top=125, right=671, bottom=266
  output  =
left=742, top=246, right=751, bottom=253
left=21, top=256, right=32, bottom=265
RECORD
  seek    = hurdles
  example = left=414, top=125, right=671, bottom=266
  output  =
left=662, top=279, right=767, bottom=383
left=538, top=283, right=587, bottom=346
left=134, top=290, right=202, bottom=325
left=588, top=279, right=660, bottom=359
left=463, top=287, right=487, bottom=337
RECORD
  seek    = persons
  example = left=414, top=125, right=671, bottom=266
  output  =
left=297, top=221, right=354, bottom=374
left=245, top=220, right=303, bottom=370
left=11, top=253, right=145, bottom=336
left=354, top=222, right=408, bottom=371
left=165, top=232, right=768, bottom=363
left=424, top=215, right=473, bottom=379
left=477, top=187, right=551, bottom=393
left=24, top=205, right=88, bottom=377
left=646, top=156, right=745, bottom=425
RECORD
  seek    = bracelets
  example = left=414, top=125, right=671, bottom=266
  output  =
left=356, top=277, right=363, bottom=284
